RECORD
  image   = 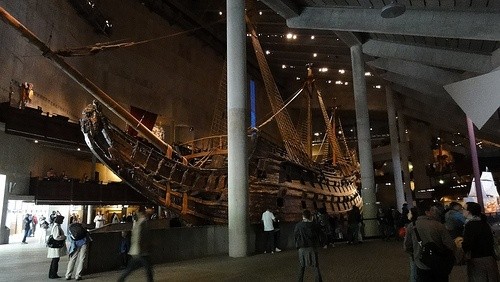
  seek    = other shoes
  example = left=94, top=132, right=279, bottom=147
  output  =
left=332, top=244, right=336, bottom=247
left=271, top=252, right=274, bottom=254
left=322, top=245, right=327, bottom=248
left=275, top=247, right=282, bottom=252
left=49, top=275, right=61, bottom=278
left=65, top=275, right=70, bottom=280
left=264, top=250, right=266, bottom=253
left=75, top=276, right=80, bottom=280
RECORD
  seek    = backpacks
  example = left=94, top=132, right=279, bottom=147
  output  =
left=68, top=223, right=87, bottom=241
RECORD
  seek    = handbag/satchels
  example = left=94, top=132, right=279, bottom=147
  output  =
left=413, top=219, right=456, bottom=278
left=47, top=225, right=65, bottom=248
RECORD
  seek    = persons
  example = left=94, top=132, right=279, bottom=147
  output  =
left=116, top=202, right=154, bottom=282
left=294, top=210, right=323, bottom=282
left=60, top=171, right=71, bottom=183
left=47, top=168, right=58, bottom=182
left=93, top=211, right=137, bottom=229
left=82, top=173, right=89, bottom=183
left=21, top=209, right=95, bottom=280
left=119, top=229, right=130, bottom=269
left=379, top=201, right=500, bottom=282
left=262, top=206, right=363, bottom=254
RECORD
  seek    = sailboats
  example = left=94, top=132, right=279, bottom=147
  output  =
left=0, top=7, right=362, bottom=224
left=462, top=166, right=500, bottom=221
